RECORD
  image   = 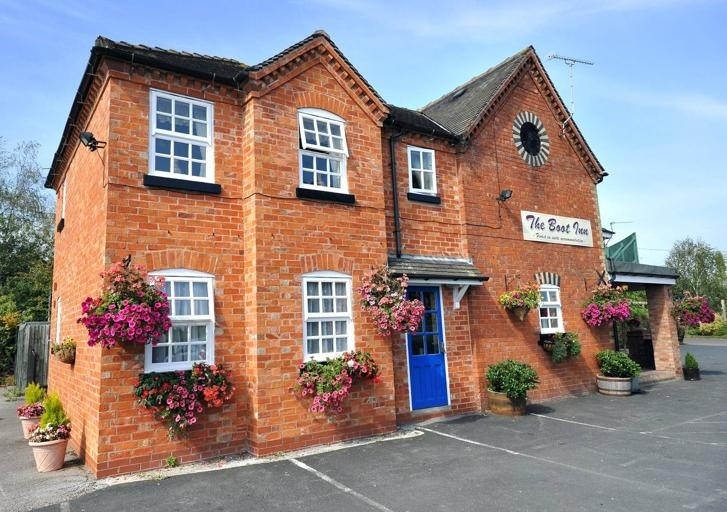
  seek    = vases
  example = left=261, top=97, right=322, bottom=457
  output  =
left=512, top=305, right=529, bottom=322
left=53, top=348, right=75, bottom=363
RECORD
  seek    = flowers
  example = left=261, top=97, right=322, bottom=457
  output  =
left=22, top=421, right=72, bottom=446
left=47, top=335, right=76, bottom=355
left=15, top=402, right=44, bottom=417
left=668, top=290, right=715, bottom=327
left=293, top=351, right=381, bottom=428
left=124, top=361, right=234, bottom=442
left=353, top=262, right=427, bottom=340
left=496, top=276, right=543, bottom=311
left=577, top=283, right=631, bottom=328
left=75, top=257, right=172, bottom=350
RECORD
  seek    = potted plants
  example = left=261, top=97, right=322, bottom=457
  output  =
left=590, top=349, right=642, bottom=396
left=542, top=329, right=581, bottom=365
left=27, top=388, right=73, bottom=473
left=15, top=379, right=48, bottom=439
left=482, top=357, right=541, bottom=417
left=681, top=351, right=700, bottom=381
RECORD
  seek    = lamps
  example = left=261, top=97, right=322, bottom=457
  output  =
left=77, top=129, right=107, bottom=153
left=495, top=188, right=513, bottom=202
